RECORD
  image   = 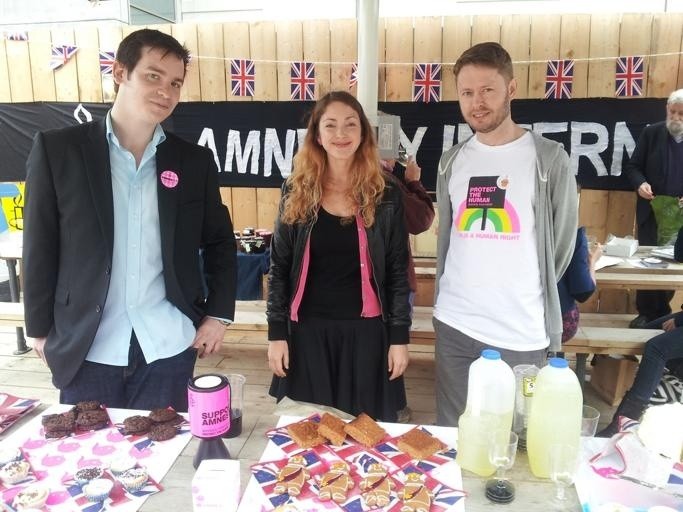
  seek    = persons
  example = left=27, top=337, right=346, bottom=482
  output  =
left=266, top=91, right=412, bottom=422
left=591, top=226, right=683, bottom=438
left=21, top=29, right=238, bottom=412
left=281, top=155, right=435, bottom=323
left=557, top=226, right=596, bottom=344
left=623, top=88, right=683, bottom=328
left=435, top=41, right=578, bottom=432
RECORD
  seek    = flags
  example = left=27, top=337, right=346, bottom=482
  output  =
left=51, top=47, right=78, bottom=71
left=232, top=60, right=256, bottom=97
left=350, top=62, right=358, bottom=90
left=98, top=51, right=117, bottom=75
left=615, top=55, right=643, bottom=97
left=1, top=391, right=194, bottom=512
left=412, top=64, right=440, bottom=102
left=246, top=411, right=470, bottom=512
left=290, top=62, right=315, bottom=100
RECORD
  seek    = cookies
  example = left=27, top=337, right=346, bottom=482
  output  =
left=316, top=462, right=355, bottom=503
left=359, top=463, right=396, bottom=506
left=398, top=473, right=435, bottom=512
left=123, top=408, right=184, bottom=441
left=273, top=455, right=311, bottom=496
left=41, top=400, right=108, bottom=437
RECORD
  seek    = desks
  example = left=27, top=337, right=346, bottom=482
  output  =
left=0, top=225, right=683, bottom=405
left=2, top=393, right=683, bottom=511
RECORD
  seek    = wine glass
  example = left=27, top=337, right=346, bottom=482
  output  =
left=547, top=442, right=584, bottom=510
left=484, top=428, right=520, bottom=504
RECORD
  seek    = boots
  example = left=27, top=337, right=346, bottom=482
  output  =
left=594, top=390, right=649, bottom=438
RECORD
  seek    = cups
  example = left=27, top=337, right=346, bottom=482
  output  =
left=584, top=234, right=599, bottom=254
left=222, top=372, right=247, bottom=439
left=579, top=403, right=600, bottom=440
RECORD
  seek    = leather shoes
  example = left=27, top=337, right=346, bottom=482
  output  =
left=629, top=315, right=649, bottom=327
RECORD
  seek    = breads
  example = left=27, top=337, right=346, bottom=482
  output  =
left=285, top=411, right=388, bottom=449
left=0, top=449, right=49, bottom=509
left=75, top=457, right=149, bottom=501
left=397, top=429, right=446, bottom=460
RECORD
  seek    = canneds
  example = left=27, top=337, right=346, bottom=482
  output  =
left=234, top=227, right=272, bottom=253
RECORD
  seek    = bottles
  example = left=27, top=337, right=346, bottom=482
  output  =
left=512, top=364, right=540, bottom=453
